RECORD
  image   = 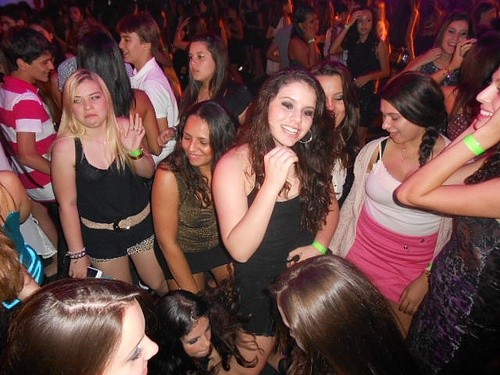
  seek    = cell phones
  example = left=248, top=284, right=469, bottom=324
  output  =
left=87, top=267, right=103, bottom=278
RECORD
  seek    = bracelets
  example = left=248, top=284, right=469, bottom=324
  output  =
left=307, top=39, right=315, bottom=43
left=464, top=134, right=484, bottom=157
left=177, top=26, right=181, bottom=30
left=344, top=25, right=349, bottom=29
left=65, top=247, right=87, bottom=259
left=426, top=263, right=433, bottom=270
left=312, top=241, right=326, bottom=255
left=442, top=67, right=450, bottom=77
left=161, top=48, right=168, bottom=52
left=129, top=146, right=143, bottom=157
left=128, top=154, right=144, bottom=161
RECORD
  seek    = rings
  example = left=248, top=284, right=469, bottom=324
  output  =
left=358, top=81, right=360, bottom=83
left=133, top=128, right=138, bottom=131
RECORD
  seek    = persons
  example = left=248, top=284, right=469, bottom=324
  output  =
left=0, top=0, right=500, bottom=375
